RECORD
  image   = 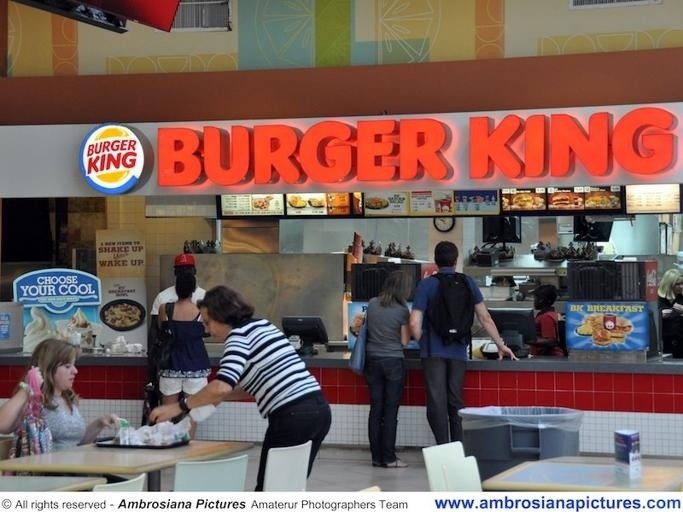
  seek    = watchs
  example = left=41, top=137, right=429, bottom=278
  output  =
left=177, top=395, right=191, bottom=414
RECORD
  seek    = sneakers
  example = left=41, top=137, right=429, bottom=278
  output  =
left=373, top=456, right=410, bottom=468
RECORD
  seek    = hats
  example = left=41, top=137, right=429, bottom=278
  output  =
left=173, top=254, right=196, bottom=268
left=528, top=284, right=557, bottom=304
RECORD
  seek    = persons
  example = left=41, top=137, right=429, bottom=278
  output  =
left=149, top=253, right=212, bottom=338
left=1, top=337, right=121, bottom=455
left=408, top=239, right=518, bottom=444
left=158, top=271, right=212, bottom=441
left=363, top=269, right=411, bottom=468
left=656, top=267, right=683, bottom=351
left=523, top=282, right=564, bottom=355
left=150, top=287, right=332, bottom=491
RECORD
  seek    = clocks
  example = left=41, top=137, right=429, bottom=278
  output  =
left=432, top=216, right=455, bottom=233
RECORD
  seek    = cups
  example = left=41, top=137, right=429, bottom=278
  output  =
left=602, top=313, right=617, bottom=332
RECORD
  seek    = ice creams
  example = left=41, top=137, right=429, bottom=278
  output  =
left=23, top=307, right=57, bottom=354
left=68, top=307, right=88, bottom=331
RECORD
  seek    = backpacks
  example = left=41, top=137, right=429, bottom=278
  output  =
left=424, top=272, right=474, bottom=346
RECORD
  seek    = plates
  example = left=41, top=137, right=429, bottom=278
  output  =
left=100, top=299, right=145, bottom=332
left=365, top=198, right=389, bottom=210
left=288, top=198, right=324, bottom=209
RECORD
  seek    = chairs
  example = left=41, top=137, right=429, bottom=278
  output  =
left=418, top=437, right=483, bottom=491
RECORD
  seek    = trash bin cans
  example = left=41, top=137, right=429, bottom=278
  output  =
left=458, top=406, right=585, bottom=491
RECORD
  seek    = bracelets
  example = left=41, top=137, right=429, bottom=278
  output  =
left=18, top=380, right=33, bottom=396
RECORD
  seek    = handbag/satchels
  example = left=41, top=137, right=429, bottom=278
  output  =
left=348, top=323, right=368, bottom=375
left=147, top=329, right=175, bottom=365
left=4, top=406, right=55, bottom=476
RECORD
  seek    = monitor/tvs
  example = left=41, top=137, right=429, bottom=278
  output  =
left=486, top=306, right=538, bottom=358
left=282, top=315, right=329, bottom=356
left=573, top=215, right=614, bottom=242
left=482, top=215, right=521, bottom=243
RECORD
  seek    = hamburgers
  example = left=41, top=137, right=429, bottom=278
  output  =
left=592, top=315, right=631, bottom=345
left=553, top=195, right=570, bottom=207
left=513, top=194, right=532, bottom=209
